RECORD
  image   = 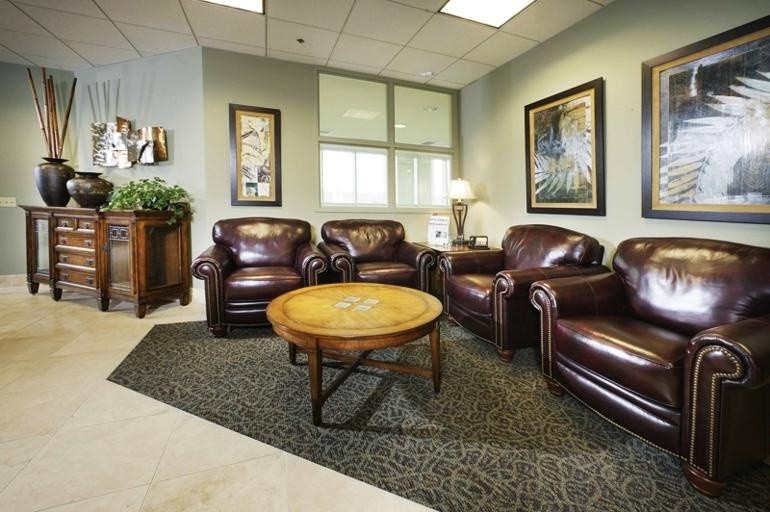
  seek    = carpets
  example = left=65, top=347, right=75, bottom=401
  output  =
left=105, top=319, right=769, bottom=511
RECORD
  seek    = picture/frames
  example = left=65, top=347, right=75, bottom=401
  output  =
left=228, top=102, right=282, bottom=207
left=524, top=77, right=605, bottom=216
left=640, top=15, right=770, bottom=225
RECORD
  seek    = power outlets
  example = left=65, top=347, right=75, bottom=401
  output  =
left=1, top=197, right=16, bottom=207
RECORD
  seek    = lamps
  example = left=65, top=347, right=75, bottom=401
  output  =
left=442, top=178, right=477, bottom=246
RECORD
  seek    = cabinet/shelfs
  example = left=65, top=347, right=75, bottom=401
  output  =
left=18, top=203, right=191, bottom=319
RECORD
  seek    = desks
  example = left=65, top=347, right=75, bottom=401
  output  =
left=412, top=241, right=500, bottom=310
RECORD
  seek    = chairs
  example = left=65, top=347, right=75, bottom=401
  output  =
left=437, top=223, right=612, bottom=363
left=317, top=218, right=437, bottom=295
left=529, top=237, right=770, bottom=498
left=190, top=216, right=328, bottom=339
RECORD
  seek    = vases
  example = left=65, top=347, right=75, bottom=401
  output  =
left=34, top=157, right=75, bottom=207
left=65, top=172, right=114, bottom=208
